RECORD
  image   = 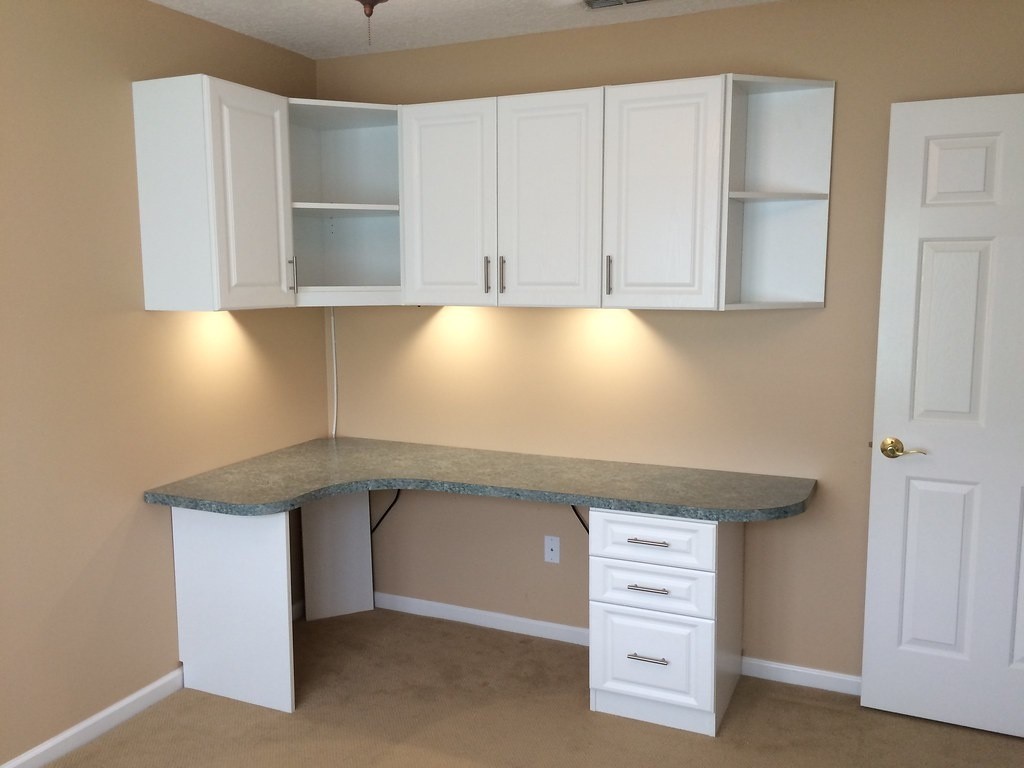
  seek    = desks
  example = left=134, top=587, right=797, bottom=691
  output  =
left=144, top=431, right=824, bottom=715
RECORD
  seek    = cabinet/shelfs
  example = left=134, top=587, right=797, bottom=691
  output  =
left=402, top=84, right=606, bottom=308
left=605, top=72, right=837, bottom=311
left=587, top=504, right=746, bottom=737
left=131, top=74, right=401, bottom=312
